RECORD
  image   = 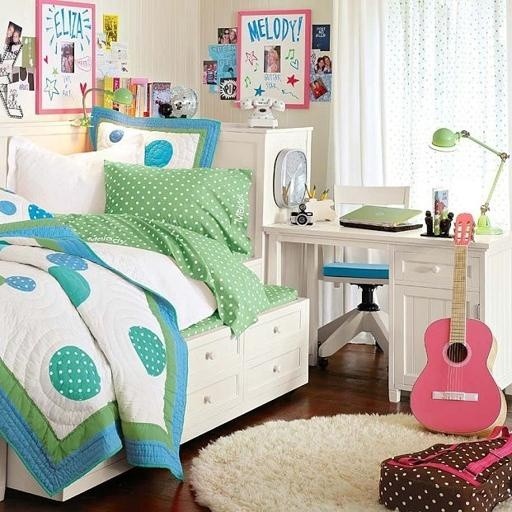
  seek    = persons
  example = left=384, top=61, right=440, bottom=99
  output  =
left=314, top=58, right=323, bottom=72
left=213, top=63, right=218, bottom=85
left=5, top=23, right=15, bottom=45
left=208, top=63, right=214, bottom=81
left=265, top=45, right=278, bottom=73
left=219, top=28, right=229, bottom=43
left=322, top=56, right=332, bottom=72
left=229, top=30, right=237, bottom=43
left=10, top=29, right=21, bottom=48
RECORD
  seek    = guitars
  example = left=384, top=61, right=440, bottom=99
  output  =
left=410, top=214, right=507, bottom=438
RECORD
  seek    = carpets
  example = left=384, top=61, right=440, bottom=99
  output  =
left=186, top=410, right=512, bottom=512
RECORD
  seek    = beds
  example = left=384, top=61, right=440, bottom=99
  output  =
left=0, top=240, right=312, bottom=503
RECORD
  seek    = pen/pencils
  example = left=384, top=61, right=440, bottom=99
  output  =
left=305, top=183, right=329, bottom=201
left=282, top=181, right=291, bottom=205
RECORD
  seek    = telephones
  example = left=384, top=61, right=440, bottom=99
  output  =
left=240, top=96, right=285, bottom=129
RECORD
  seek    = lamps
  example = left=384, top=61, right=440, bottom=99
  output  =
left=64, top=82, right=134, bottom=128
left=428, top=127, right=510, bottom=235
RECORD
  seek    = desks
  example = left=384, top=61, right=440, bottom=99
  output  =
left=259, top=218, right=511, bottom=404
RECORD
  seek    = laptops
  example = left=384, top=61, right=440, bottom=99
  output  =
left=338, top=205, right=423, bottom=232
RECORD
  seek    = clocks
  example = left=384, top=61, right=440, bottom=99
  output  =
left=271, top=142, right=310, bottom=209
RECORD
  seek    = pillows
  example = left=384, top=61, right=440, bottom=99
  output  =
left=101, top=157, right=255, bottom=263
left=4, top=129, right=146, bottom=219
left=87, top=101, right=221, bottom=170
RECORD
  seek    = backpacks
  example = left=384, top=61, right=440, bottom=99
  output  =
left=377, top=423, right=512, bottom=511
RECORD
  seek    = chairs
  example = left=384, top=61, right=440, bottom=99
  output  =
left=315, top=182, right=412, bottom=369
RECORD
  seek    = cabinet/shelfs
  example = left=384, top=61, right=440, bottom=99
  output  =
left=210, top=122, right=317, bottom=300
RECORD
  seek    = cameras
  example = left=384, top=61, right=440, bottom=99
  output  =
left=290, top=204, right=313, bottom=226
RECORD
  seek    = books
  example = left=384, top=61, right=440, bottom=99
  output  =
left=103, top=75, right=172, bottom=119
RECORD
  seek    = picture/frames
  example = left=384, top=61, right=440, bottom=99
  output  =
left=234, top=9, right=312, bottom=110
left=34, top=0, right=99, bottom=117
